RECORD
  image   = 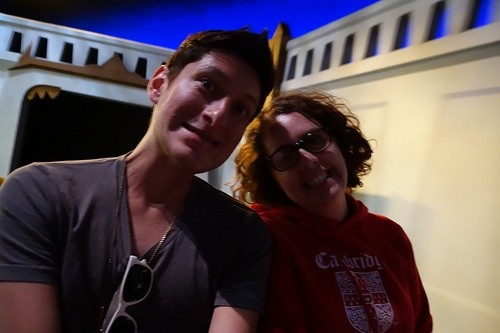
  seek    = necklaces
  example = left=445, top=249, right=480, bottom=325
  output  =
left=97, top=160, right=192, bottom=332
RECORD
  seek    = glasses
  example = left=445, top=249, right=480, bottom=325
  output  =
left=100, top=255, right=153, bottom=333
left=266, top=125, right=332, bottom=172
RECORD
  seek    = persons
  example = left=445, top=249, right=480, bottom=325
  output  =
left=232, top=89, right=434, bottom=333
left=0, top=28, right=277, bottom=333
